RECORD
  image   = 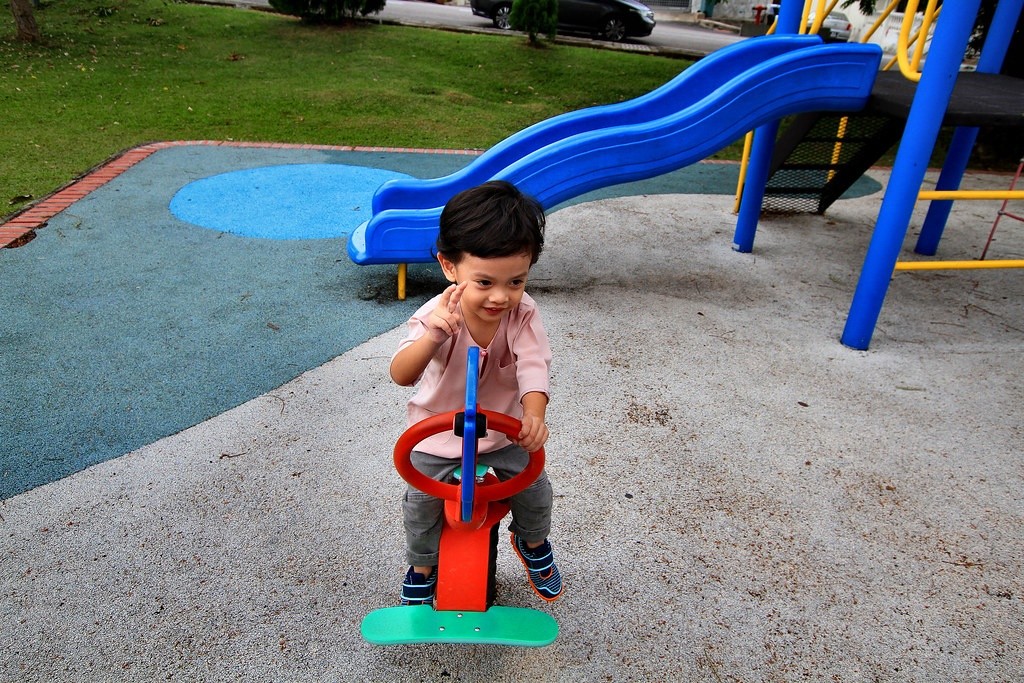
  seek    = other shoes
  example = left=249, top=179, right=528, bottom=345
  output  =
left=401, top=565, right=438, bottom=602
left=511, top=530, right=563, bottom=602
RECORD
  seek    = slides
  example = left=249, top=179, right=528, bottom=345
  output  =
left=348, top=33, right=883, bottom=266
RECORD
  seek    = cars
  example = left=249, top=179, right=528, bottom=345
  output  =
left=469, top=0, right=656, bottom=42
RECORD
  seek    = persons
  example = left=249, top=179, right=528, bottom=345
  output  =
left=390, top=181, right=563, bottom=609
